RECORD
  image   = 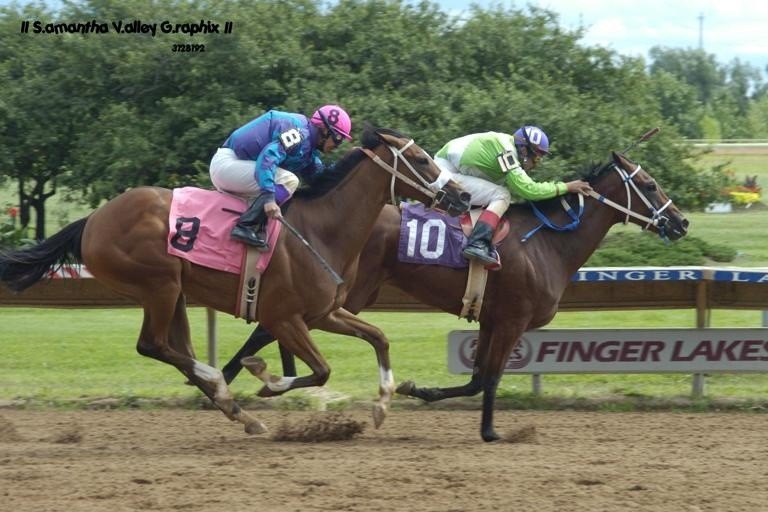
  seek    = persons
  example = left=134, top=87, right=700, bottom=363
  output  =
left=205, top=102, right=353, bottom=253
left=433, top=123, right=594, bottom=269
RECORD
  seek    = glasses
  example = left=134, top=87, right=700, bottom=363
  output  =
left=529, top=150, right=543, bottom=163
left=331, top=131, right=343, bottom=145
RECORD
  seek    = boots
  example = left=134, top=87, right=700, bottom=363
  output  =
left=462, top=208, right=503, bottom=271
left=230, top=190, right=275, bottom=252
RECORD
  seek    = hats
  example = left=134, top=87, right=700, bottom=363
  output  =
left=512, top=125, right=552, bottom=156
left=311, top=104, right=353, bottom=140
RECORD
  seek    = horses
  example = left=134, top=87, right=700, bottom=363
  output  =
left=1, top=116, right=474, bottom=434
left=183, top=149, right=692, bottom=442
